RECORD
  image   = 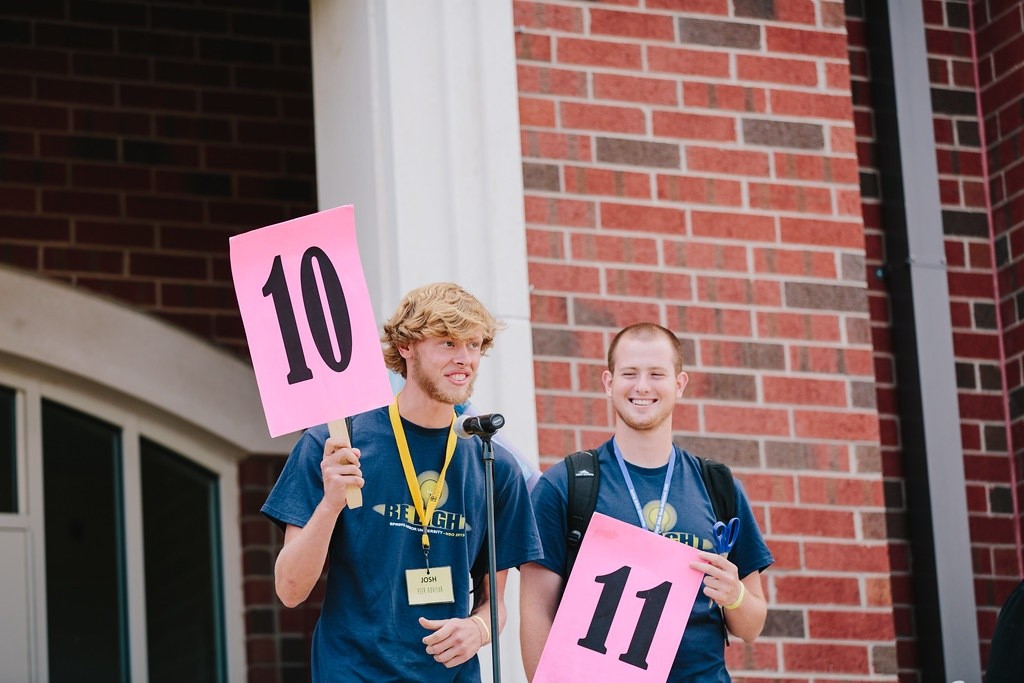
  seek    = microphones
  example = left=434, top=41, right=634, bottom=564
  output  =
left=453, top=414, right=505, bottom=440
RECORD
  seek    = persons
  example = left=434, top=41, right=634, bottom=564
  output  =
left=515, top=323, right=775, bottom=683
left=260, top=282, right=545, bottom=683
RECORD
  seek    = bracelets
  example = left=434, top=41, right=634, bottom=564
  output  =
left=470, top=614, right=490, bottom=645
left=724, top=580, right=745, bottom=610
